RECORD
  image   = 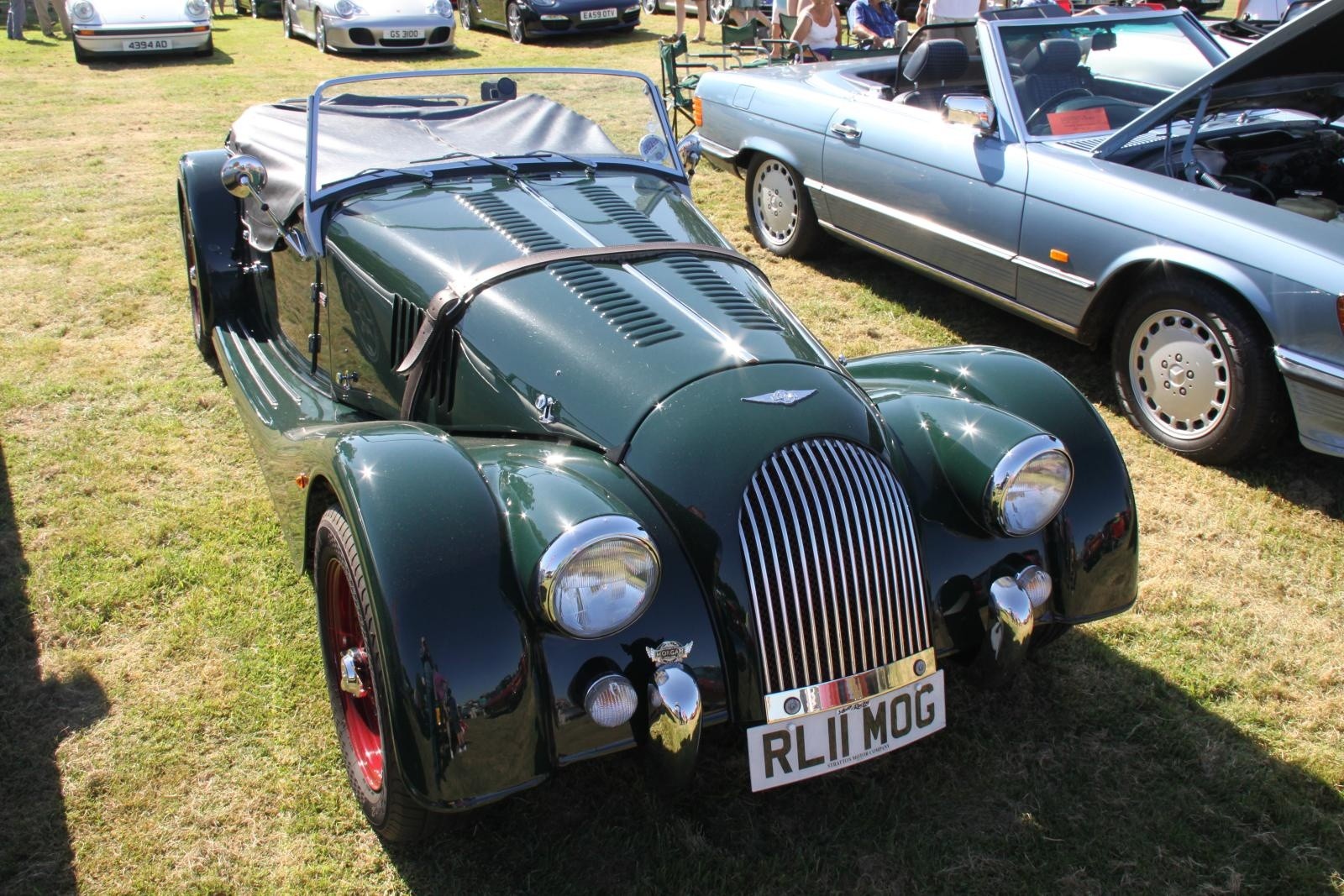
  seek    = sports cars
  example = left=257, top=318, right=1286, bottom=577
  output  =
left=693, top=0, right=1343, bottom=465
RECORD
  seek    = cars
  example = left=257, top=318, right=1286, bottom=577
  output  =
left=637, top=0, right=775, bottom=25
left=232, top=0, right=290, bottom=21
left=61, top=0, right=216, bottom=63
left=457, top=0, right=642, bottom=44
left=279, top=0, right=457, bottom=54
left=174, top=63, right=1136, bottom=845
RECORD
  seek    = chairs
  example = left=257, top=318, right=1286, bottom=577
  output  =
left=1013, top=39, right=1087, bottom=122
left=661, top=8, right=874, bottom=146
left=891, top=38, right=975, bottom=112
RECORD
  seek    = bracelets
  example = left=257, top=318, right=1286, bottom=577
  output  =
left=920, top=3, right=927, bottom=8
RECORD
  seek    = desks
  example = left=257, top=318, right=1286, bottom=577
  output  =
left=831, top=46, right=893, bottom=61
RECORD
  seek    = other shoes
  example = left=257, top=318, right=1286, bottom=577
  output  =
left=766, top=55, right=779, bottom=60
left=689, top=36, right=705, bottom=43
left=661, top=34, right=677, bottom=43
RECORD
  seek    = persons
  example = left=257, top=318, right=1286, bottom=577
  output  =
left=790, top=0, right=843, bottom=63
left=7, top=0, right=28, bottom=40
left=847, top=0, right=899, bottom=49
left=34, top=0, right=72, bottom=37
left=210, top=0, right=225, bottom=15
left=1236, top=0, right=1288, bottom=20
left=662, top=0, right=707, bottom=42
left=916, top=0, right=987, bottom=53
left=730, top=0, right=811, bottom=59
